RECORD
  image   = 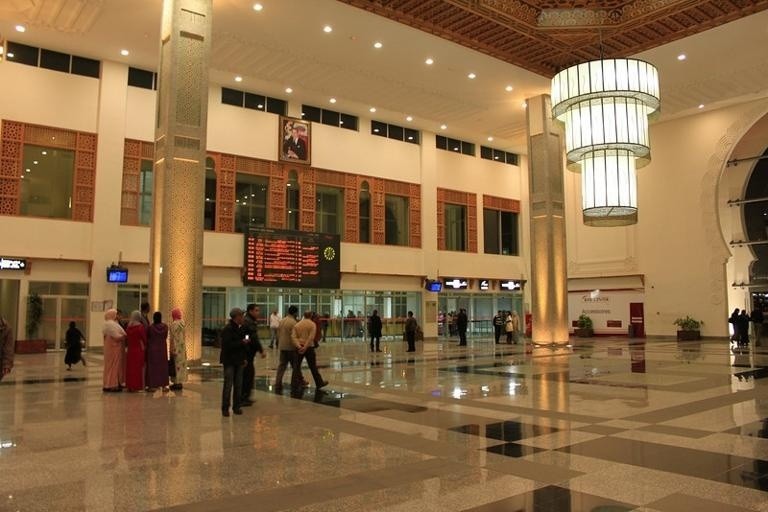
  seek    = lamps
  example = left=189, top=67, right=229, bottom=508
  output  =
left=549, top=0, right=660, bottom=228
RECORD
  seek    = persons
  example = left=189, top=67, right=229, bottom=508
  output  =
left=730, top=344, right=763, bottom=383
left=274, top=305, right=310, bottom=389
left=64, top=321, right=87, bottom=371
left=238, top=303, right=266, bottom=406
left=493, top=309, right=519, bottom=344
left=268, top=303, right=364, bottom=349
left=104, top=302, right=187, bottom=393
left=96, top=389, right=339, bottom=494
left=728, top=306, right=763, bottom=348
left=404, top=311, right=416, bottom=352
left=367, top=310, right=382, bottom=352
left=218, top=307, right=251, bottom=416
left=290, top=310, right=328, bottom=389
left=367, top=347, right=527, bottom=392
left=437, top=307, right=468, bottom=345
left=283, top=120, right=306, bottom=161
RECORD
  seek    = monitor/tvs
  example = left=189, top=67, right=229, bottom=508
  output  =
left=107, top=265, right=128, bottom=282
left=426, top=279, right=442, bottom=292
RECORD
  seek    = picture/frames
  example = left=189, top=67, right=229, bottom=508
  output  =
left=277, top=112, right=315, bottom=166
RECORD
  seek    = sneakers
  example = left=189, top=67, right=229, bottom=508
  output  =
left=276, top=377, right=329, bottom=392
left=221, top=397, right=255, bottom=417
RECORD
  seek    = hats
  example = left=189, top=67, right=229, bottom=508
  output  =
left=230, top=308, right=248, bottom=317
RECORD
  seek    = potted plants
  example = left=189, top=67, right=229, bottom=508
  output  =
left=674, top=315, right=703, bottom=341
left=13, top=292, right=49, bottom=352
left=576, top=311, right=593, bottom=337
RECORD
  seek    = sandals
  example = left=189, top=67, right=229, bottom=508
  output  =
left=145, top=387, right=155, bottom=392
left=162, top=387, right=170, bottom=392
